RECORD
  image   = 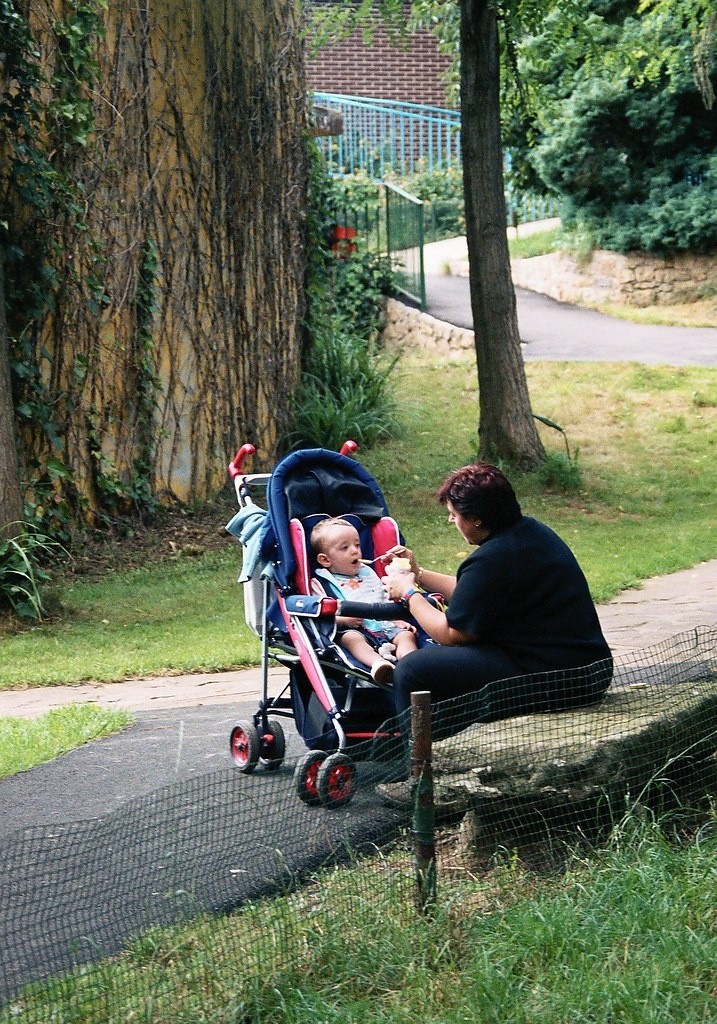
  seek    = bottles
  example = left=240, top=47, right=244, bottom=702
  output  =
left=392, top=557, right=411, bottom=576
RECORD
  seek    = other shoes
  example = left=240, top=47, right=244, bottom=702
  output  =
left=374, top=775, right=418, bottom=811
left=371, top=660, right=394, bottom=685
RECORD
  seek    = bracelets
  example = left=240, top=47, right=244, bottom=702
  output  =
left=417, top=567, right=423, bottom=587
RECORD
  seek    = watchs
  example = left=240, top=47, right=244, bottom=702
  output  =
left=400, top=587, right=420, bottom=609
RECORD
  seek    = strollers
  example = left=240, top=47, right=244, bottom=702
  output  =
left=227, top=440, right=442, bottom=804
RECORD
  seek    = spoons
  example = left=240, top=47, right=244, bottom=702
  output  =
left=357, top=549, right=405, bottom=564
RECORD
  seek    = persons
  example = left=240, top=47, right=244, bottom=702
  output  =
left=376, top=463, right=613, bottom=807
left=310, top=518, right=419, bottom=682
